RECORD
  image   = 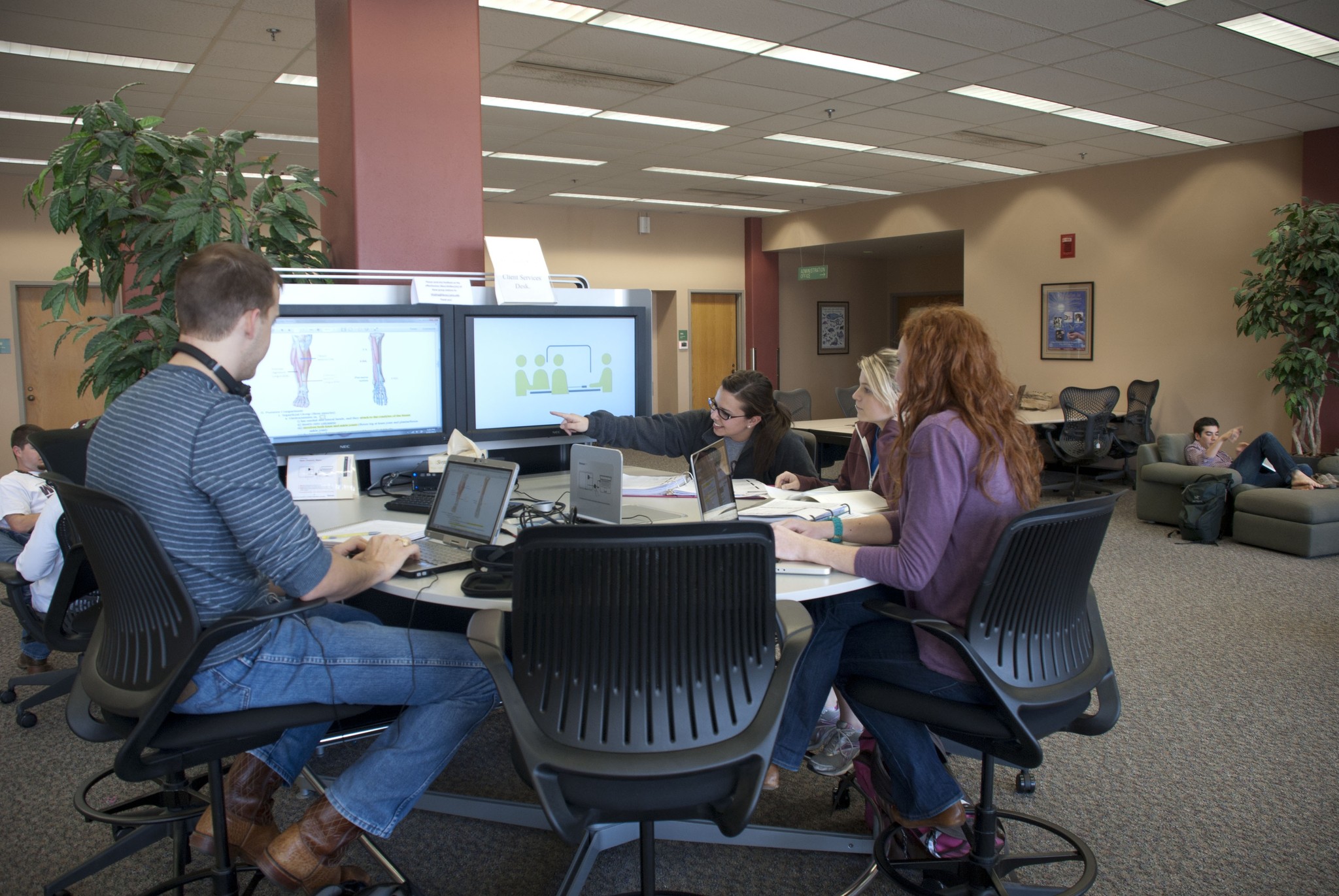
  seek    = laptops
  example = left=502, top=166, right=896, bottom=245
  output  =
left=569, top=443, right=688, bottom=524
left=347, top=455, right=520, bottom=579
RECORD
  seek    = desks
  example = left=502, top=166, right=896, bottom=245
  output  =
left=788, top=406, right=1128, bottom=480
left=249, top=466, right=896, bottom=618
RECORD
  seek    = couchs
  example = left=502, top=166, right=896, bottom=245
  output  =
left=1135, top=431, right=1339, bottom=558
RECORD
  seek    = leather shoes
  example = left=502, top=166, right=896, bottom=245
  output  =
left=895, top=800, right=965, bottom=828
left=762, top=765, right=780, bottom=790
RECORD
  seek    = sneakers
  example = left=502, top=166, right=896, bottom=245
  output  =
left=807, top=720, right=863, bottom=777
left=805, top=702, right=841, bottom=751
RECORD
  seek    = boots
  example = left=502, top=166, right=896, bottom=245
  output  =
left=256, top=793, right=373, bottom=896
left=189, top=753, right=283, bottom=861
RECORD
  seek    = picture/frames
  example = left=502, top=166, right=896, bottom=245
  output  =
left=817, top=301, right=850, bottom=355
left=1040, top=282, right=1094, bottom=361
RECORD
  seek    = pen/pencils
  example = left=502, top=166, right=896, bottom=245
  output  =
left=321, top=531, right=380, bottom=539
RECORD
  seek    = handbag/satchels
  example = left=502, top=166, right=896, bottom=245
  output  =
left=846, top=722, right=1005, bottom=859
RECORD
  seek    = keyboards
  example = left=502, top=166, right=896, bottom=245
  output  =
left=383, top=490, right=523, bottom=516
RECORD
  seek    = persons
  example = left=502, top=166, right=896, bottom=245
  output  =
left=774, top=347, right=912, bottom=776
left=762, top=305, right=1044, bottom=826
left=1184, top=417, right=1323, bottom=491
left=0, top=424, right=64, bottom=674
left=549, top=369, right=820, bottom=485
left=86, top=242, right=511, bottom=896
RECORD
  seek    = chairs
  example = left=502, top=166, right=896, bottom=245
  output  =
left=0, top=379, right=1163, bottom=896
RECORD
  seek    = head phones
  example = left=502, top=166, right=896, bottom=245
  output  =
left=171, top=341, right=253, bottom=405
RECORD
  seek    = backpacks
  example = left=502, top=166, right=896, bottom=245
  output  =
left=1176, top=472, right=1234, bottom=545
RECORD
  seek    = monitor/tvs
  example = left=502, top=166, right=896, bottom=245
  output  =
left=690, top=439, right=834, bottom=576
left=454, top=305, right=647, bottom=442
left=241, top=304, right=455, bottom=456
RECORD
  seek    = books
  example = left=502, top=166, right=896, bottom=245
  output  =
left=738, top=498, right=850, bottom=524
left=767, top=485, right=890, bottom=513
left=621, top=472, right=768, bottom=499
left=315, top=519, right=427, bottom=549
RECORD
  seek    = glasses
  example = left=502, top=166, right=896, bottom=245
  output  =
left=707, top=397, right=747, bottom=420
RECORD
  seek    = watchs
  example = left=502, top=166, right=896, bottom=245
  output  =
left=823, top=515, right=842, bottom=542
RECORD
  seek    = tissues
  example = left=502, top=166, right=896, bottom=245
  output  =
left=428, top=428, right=488, bottom=473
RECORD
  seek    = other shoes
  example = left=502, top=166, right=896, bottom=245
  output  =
left=1311, top=475, right=1337, bottom=489
left=18, top=652, right=53, bottom=674
left=1315, top=473, right=1339, bottom=488
